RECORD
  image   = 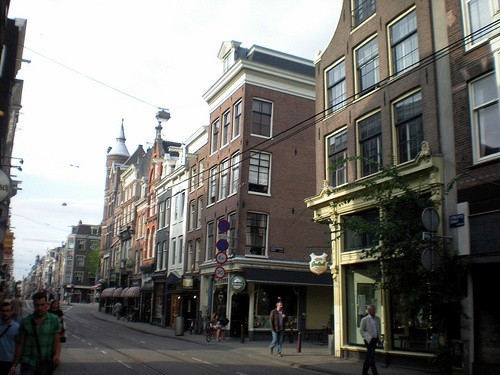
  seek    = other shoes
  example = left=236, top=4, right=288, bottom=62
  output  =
left=268, top=347, right=273, bottom=355
left=277, top=352, right=281, bottom=357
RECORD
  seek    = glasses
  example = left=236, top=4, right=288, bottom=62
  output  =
left=34, top=302, right=46, bottom=306
left=1, top=309, right=12, bottom=312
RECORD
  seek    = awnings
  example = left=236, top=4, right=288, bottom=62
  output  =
left=101, top=286, right=140, bottom=298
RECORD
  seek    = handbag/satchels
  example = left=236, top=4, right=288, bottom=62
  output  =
left=36, top=359, right=54, bottom=375
left=220, top=316, right=229, bottom=327
left=8, top=361, right=21, bottom=375
left=60, top=336, right=66, bottom=343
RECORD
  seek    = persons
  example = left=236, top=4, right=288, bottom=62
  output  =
left=12, top=291, right=62, bottom=375
left=11, top=294, right=33, bottom=320
left=360, top=304, right=381, bottom=375
left=114, top=303, right=122, bottom=319
left=329, top=314, right=334, bottom=334
left=270, top=302, right=289, bottom=357
left=0, top=302, right=20, bottom=375
left=47, top=300, right=66, bottom=363
left=214, top=306, right=229, bottom=342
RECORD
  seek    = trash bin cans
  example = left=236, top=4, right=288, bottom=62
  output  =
left=174, top=316, right=185, bottom=336
left=328, top=334, right=335, bottom=355
